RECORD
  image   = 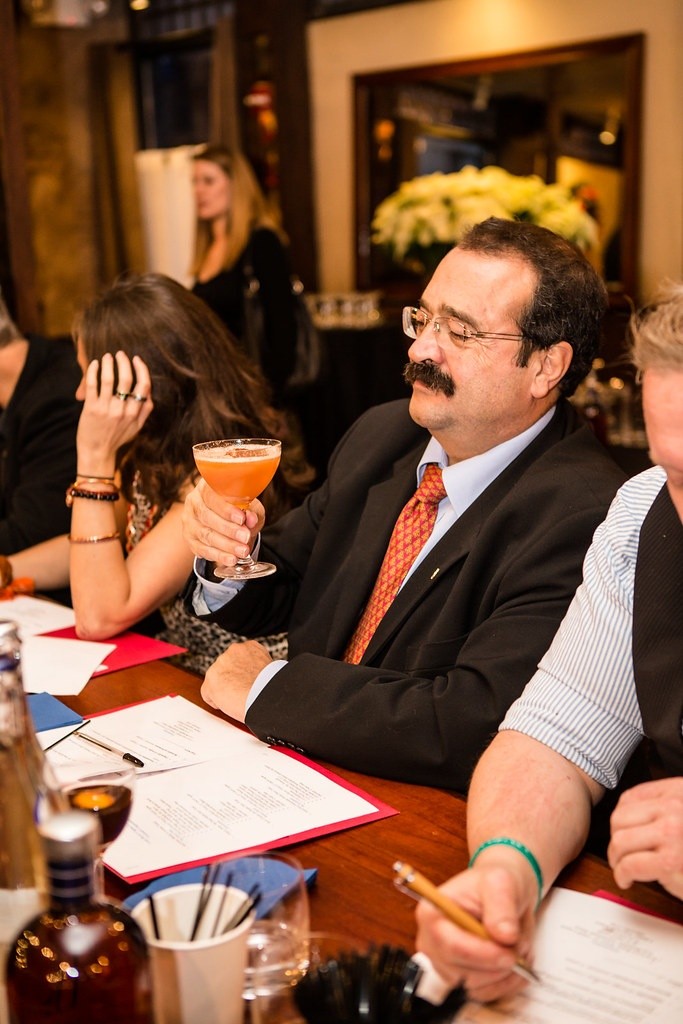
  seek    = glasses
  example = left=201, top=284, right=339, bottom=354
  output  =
left=398, top=304, right=522, bottom=350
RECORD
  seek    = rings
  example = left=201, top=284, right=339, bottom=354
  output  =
left=113, top=390, right=129, bottom=400
left=130, top=394, right=147, bottom=402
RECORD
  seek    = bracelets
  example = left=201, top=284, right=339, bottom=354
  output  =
left=468, top=838, right=543, bottom=909
left=66, top=472, right=122, bottom=544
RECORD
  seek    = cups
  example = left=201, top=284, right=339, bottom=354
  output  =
left=571, top=383, right=647, bottom=448
left=130, top=883, right=256, bottom=1024
left=251, top=933, right=369, bottom=1024
left=307, top=292, right=374, bottom=327
left=202, top=848, right=312, bottom=999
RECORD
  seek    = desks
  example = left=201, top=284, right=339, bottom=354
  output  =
left=57, top=659, right=683, bottom=1024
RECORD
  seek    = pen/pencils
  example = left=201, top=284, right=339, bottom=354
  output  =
left=73, top=730, right=144, bottom=767
left=391, top=861, right=539, bottom=983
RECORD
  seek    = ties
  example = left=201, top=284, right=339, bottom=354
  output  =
left=346, top=461, right=448, bottom=666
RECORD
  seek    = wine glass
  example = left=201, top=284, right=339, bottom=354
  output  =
left=40, top=749, right=136, bottom=909
left=191, top=436, right=283, bottom=579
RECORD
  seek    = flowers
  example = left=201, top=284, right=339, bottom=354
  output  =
left=366, top=160, right=602, bottom=274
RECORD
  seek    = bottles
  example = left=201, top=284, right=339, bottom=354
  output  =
left=581, top=372, right=610, bottom=447
left=4, top=810, right=157, bottom=1024
left=0, top=621, right=45, bottom=892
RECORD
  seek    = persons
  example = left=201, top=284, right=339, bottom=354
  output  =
left=180, top=214, right=636, bottom=798
left=187, top=144, right=297, bottom=409
left=411, top=276, right=683, bottom=1004
left=0, top=304, right=85, bottom=612
left=0, top=273, right=313, bottom=678
